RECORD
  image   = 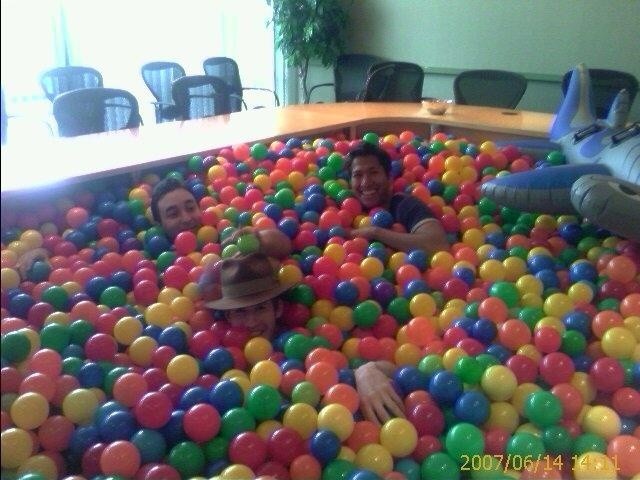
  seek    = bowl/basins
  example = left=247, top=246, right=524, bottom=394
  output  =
left=423, top=98, right=453, bottom=115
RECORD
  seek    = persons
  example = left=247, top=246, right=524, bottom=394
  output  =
left=205, top=251, right=405, bottom=427
left=345, top=141, right=450, bottom=251
left=150, top=177, right=292, bottom=255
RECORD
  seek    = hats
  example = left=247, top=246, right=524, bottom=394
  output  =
left=201, top=249, right=293, bottom=313
